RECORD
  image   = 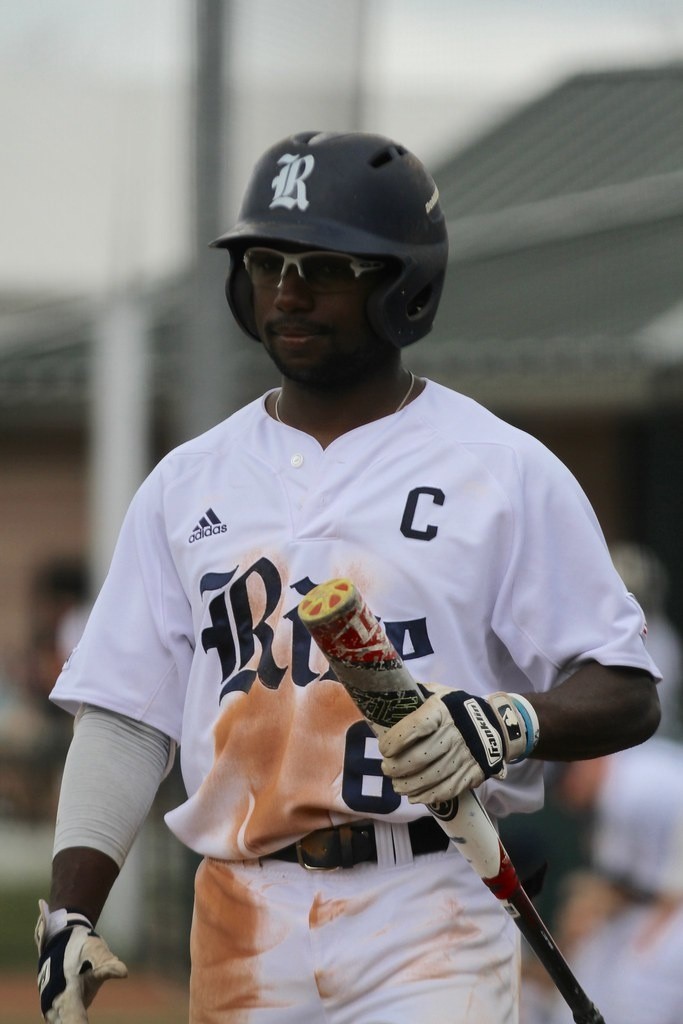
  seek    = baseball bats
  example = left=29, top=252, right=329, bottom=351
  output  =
left=294, top=578, right=609, bottom=1024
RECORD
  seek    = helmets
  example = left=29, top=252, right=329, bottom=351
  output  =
left=207, top=132, right=448, bottom=350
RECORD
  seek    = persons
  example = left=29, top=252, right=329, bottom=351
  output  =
left=28, top=553, right=89, bottom=766
left=34, top=134, right=682, bottom=1024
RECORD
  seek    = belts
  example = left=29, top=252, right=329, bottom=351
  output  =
left=259, top=816, right=450, bottom=871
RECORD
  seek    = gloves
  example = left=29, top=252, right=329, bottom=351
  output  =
left=33, top=899, right=128, bottom=1024
left=378, top=681, right=539, bottom=805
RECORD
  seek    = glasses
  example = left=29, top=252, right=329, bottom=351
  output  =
left=242, top=248, right=391, bottom=296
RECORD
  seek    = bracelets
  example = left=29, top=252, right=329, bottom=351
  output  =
left=507, top=692, right=539, bottom=752
left=510, top=698, right=532, bottom=764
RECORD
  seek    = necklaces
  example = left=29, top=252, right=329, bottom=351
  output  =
left=275, top=370, right=415, bottom=423
